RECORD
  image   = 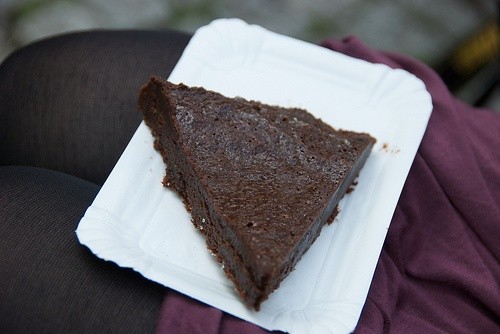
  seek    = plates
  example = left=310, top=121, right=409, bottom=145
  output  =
left=75, top=18, right=435, bottom=334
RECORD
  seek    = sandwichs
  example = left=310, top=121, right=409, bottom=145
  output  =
left=137, top=76, right=376, bottom=310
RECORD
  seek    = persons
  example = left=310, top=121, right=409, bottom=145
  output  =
left=0, top=27, right=499, bottom=334
